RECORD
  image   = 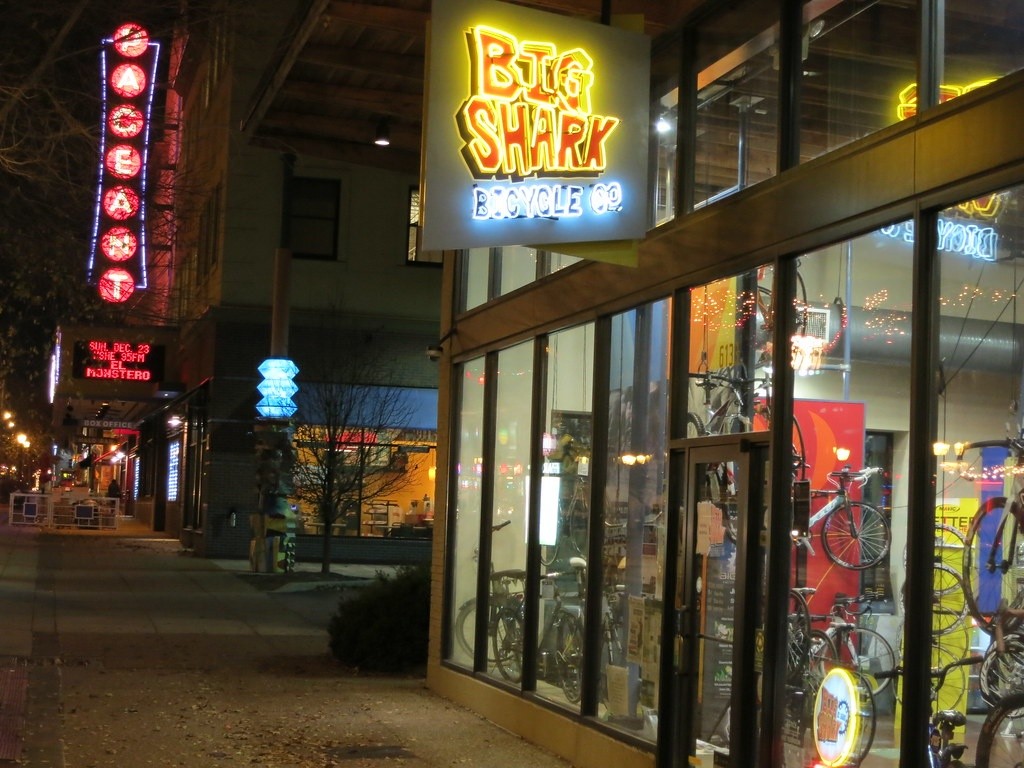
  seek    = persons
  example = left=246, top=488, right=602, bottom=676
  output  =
left=108, top=478, right=122, bottom=514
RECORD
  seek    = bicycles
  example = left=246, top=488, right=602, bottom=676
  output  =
left=452, top=338, right=1023, bottom=767
